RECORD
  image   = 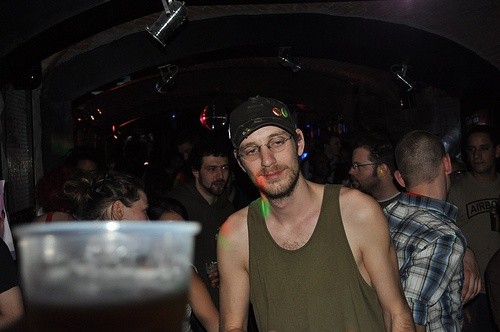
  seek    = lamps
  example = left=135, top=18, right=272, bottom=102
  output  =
left=390, top=63, right=414, bottom=93
left=277, top=46, right=303, bottom=73
left=155, top=64, right=179, bottom=95
left=143, top=0, right=189, bottom=48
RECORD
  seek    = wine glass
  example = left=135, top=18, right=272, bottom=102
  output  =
left=205, top=258, right=220, bottom=289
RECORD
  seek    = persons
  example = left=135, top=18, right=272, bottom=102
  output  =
left=445, top=158, right=464, bottom=185
left=445, top=123, right=500, bottom=332
left=216, top=96, right=416, bottom=332
left=0, top=114, right=408, bottom=332
left=382, top=130, right=482, bottom=332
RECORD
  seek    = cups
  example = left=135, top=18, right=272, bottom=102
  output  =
left=12, top=219, right=202, bottom=332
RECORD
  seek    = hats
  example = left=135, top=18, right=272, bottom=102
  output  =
left=229, top=97, right=296, bottom=147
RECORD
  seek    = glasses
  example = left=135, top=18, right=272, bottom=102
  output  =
left=349, top=161, right=381, bottom=171
left=239, top=135, right=292, bottom=162
